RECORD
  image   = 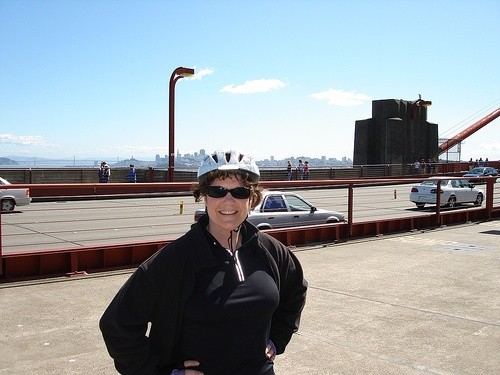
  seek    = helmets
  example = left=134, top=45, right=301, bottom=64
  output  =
left=197, top=150, right=260, bottom=179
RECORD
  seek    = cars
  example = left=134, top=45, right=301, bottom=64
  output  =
left=409, top=176, right=485, bottom=208
left=194, top=192, right=347, bottom=230
left=0, top=176, right=31, bottom=211
left=463, top=167, right=498, bottom=183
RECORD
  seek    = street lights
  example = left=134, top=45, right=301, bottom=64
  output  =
left=410, top=99, right=432, bottom=162
left=168, top=67, right=195, bottom=181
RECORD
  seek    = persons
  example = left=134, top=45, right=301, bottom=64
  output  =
left=125, top=164, right=137, bottom=183
left=98, top=162, right=110, bottom=183
left=468, top=157, right=488, bottom=170
left=286, top=160, right=292, bottom=180
left=413, top=158, right=437, bottom=174
left=304, top=161, right=309, bottom=179
left=296, top=159, right=304, bottom=180
left=99, top=149, right=308, bottom=375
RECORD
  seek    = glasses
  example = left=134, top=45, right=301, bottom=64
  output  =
left=207, top=186, right=250, bottom=199
